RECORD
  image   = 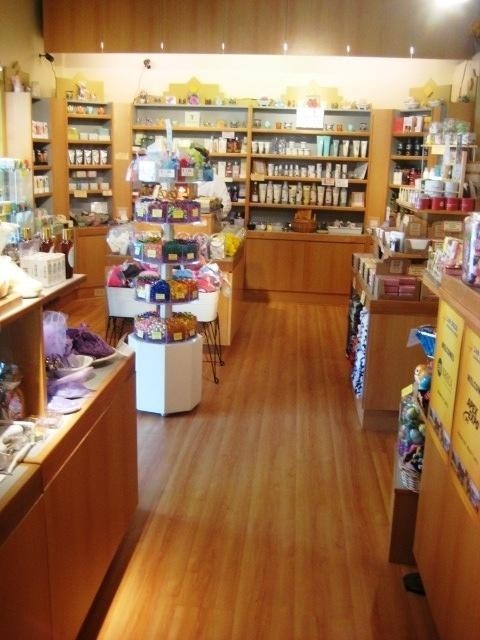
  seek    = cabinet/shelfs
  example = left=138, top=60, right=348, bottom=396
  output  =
left=128, top=133, right=207, bottom=418
left=0, top=273, right=139, bottom=640
left=122, top=86, right=251, bottom=346
left=243, top=90, right=372, bottom=294
left=346, top=88, right=476, bottom=434
left=5, top=87, right=124, bottom=291
left=392, top=261, right=480, bottom=637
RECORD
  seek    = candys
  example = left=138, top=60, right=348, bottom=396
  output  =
left=135, top=275, right=198, bottom=303
left=134, top=311, right=197, bottom=343
left=135, top=196, right=200, bottom=224
left=134, top=236, right=198, bottom=265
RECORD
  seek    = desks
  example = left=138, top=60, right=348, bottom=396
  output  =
left=103, top=285, right=225, bottom=387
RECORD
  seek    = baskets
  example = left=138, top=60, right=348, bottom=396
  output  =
left=291, top=218, right=318, bottom=233
